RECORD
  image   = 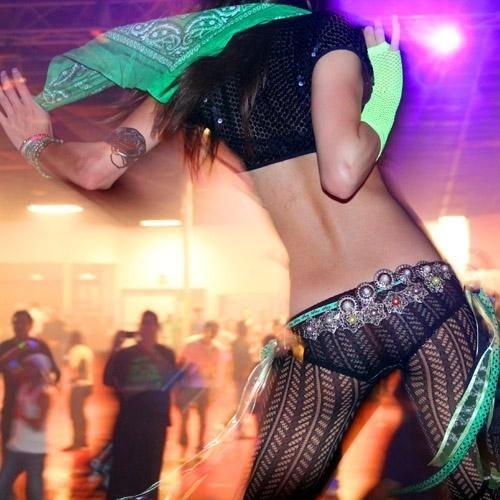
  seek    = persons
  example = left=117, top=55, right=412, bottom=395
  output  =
left=0, top=0, right=498, bottom=500
left=1, top=311, right=285, bottom=500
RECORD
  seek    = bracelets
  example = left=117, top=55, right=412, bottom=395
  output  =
left=18, top=132, right=64, bottom=178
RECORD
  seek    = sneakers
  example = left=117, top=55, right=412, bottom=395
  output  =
left=179, top=441, right=205, bottom=450
left=61, top=444, right=89, bottom=452
